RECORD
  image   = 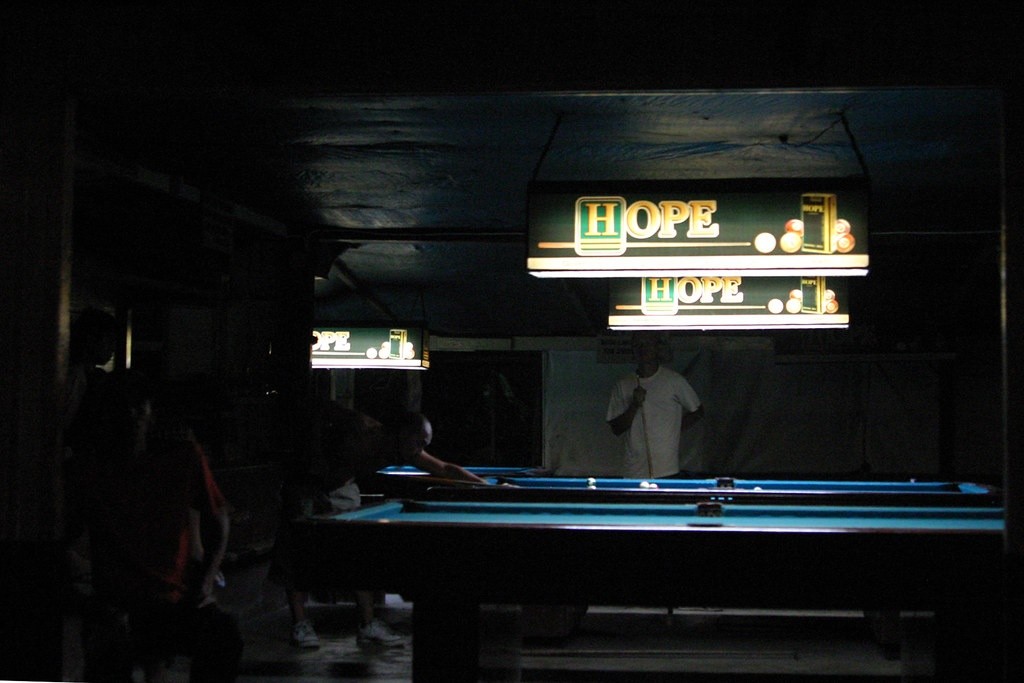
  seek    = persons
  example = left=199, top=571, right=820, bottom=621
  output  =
left=606, top=330, right=706, bottom=479
left=65, top=306, right=254, bottom=683
left=267, top=396, right=500, bottom=647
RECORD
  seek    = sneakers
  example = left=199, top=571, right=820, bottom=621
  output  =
left=355, top=621, right=413, bottom=648
left=289, top=618, right=323, bottom=650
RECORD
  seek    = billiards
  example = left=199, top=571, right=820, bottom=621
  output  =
left=587, top=478, right=762, bottom=491
left=768, top=289, right=838, bottom=314
left=366, top=341, right=415, bottom=359
left=754, top=218, right=856, bottom=254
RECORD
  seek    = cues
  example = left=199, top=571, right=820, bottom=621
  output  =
left=538, top=242, right=752, bottom=248
left=312, top=351, right=365, bottom=355
left=616, top=305, right=766, bottom=310
left=635, top=375, right=653, bottom=479
left=380, top=475, right=524, bottom=489
